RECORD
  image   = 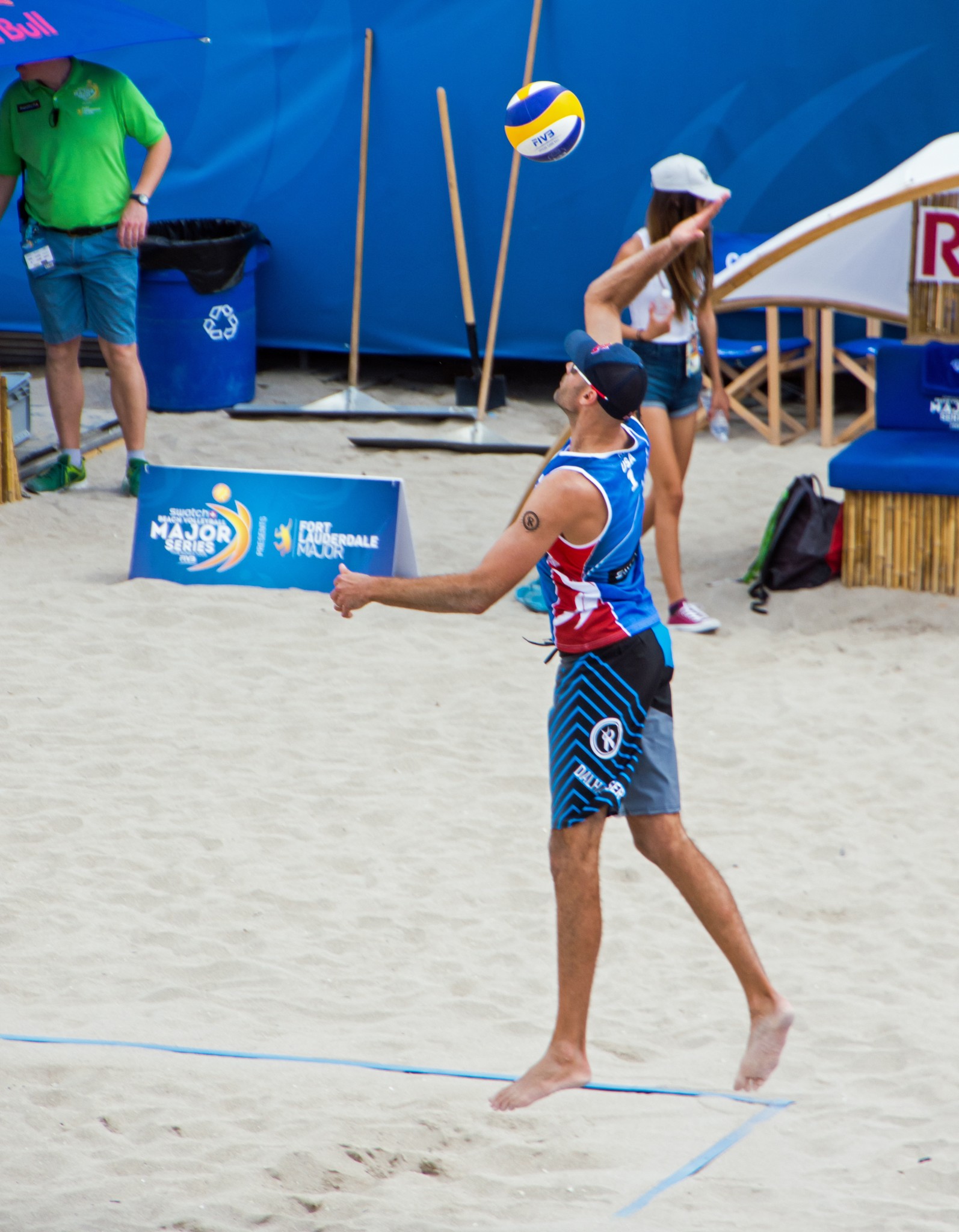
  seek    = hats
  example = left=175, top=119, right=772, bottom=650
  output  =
left=565, top=328, right=648, bottom=419
left=650, top=151, right=731, bottom=203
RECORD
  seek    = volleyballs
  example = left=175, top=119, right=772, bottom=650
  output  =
left=503, top=80, right=584, bottom=162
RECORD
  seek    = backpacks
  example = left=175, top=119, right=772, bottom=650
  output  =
left=738, top=473, right=843, bottom=591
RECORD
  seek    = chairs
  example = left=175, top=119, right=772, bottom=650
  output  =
left=703, top=304, right=881, bottom=446
left=828, top=333, right=959, bottom=600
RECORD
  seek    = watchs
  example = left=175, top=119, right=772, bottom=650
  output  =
left=130, top=192, right=150, bottom=206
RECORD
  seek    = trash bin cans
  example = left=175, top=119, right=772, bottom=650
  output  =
left=138, top=217, right=262, bottom=413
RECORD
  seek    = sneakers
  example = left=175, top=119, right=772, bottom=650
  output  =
left=124, top=458, right=151, bottom=498
left=667, top=598, right=721, bottom=631
left=25, top=452, right=88, bottom=495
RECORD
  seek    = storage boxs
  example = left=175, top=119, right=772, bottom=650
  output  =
left=0, top=371, right=31, bottom=445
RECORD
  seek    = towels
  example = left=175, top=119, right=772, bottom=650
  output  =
left=918, top=338, right=959, bottom=399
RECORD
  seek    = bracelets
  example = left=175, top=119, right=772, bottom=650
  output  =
left=637, top=328, right=645, bottom=341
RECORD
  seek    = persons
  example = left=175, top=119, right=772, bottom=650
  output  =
left=0, top=56, right=171, bottom=499
left=611, top=152, right=731, bottom=633
left=329, top=196, right=792, bottom=1112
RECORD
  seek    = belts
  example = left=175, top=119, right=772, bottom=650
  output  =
left=37, top=222, right=119, bottom=237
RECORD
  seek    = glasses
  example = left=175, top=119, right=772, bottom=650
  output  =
left=570, top=363, right=608, bottom=400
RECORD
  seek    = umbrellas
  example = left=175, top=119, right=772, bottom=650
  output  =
left=0, top=0, right=210, bottom=66
